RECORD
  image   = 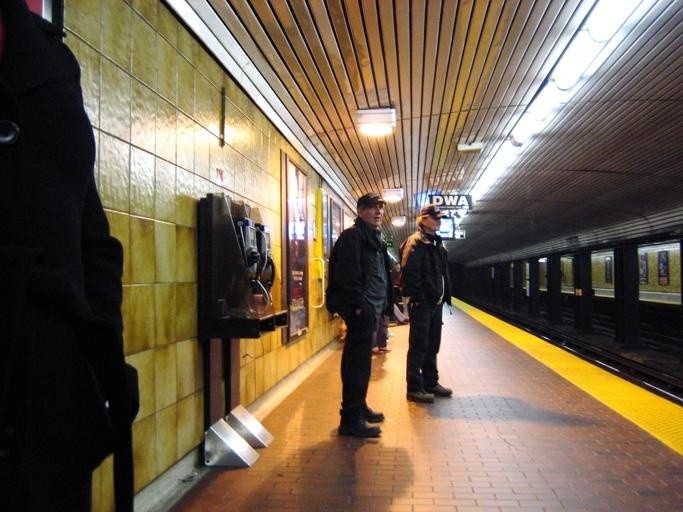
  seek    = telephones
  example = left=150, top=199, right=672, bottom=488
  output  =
left=237, top=217, right=275, bottom=295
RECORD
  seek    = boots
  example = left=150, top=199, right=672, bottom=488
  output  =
left=426, top=384, right=453, bottom=397
left=406, top=389, right=435, bottom=403
left=338, top=401, right=385, bottom=438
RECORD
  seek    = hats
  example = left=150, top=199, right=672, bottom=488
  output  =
left=358, top=193, right=389, bottom=209
left=420, top=204, right=449, bottom=221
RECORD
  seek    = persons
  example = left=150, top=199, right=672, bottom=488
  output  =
left=0, top=0, right=129, bottom=512
left=397, top=204, right=453, bottom=403
left=325, top=191, right=409, bottom=437
